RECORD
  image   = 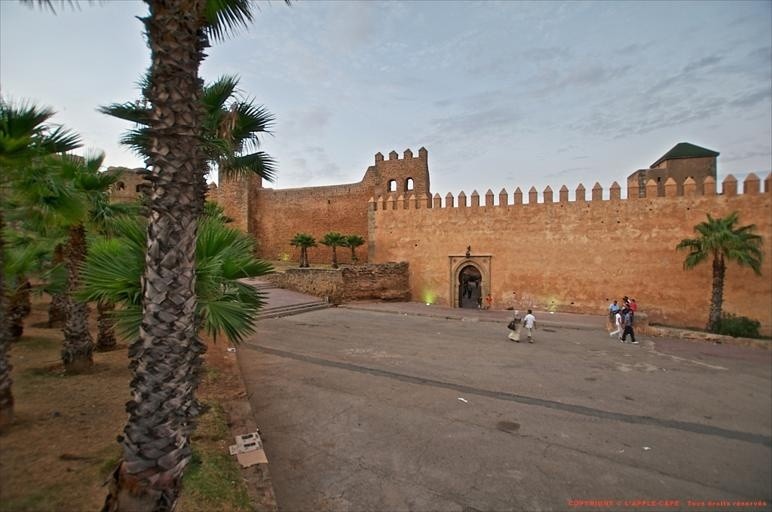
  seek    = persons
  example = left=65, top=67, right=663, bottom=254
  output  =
left=478, top=296, right=483, bottom=311
left=508, top=309, right=522, bottom=342
left=522, top=310, right=537, bottom=343
left=467, top=284, right=472, bottom=298
left=483, top=294, right=493, bottom=310
left=608, top=295, right=637, bottom=329
left=609, top=309, right=624, bottom=340
left=620, top=309, right=638, bottom=344
left=475, top=279, right=479, bottom=288
left=462, top=278, right=468, bottom=296
